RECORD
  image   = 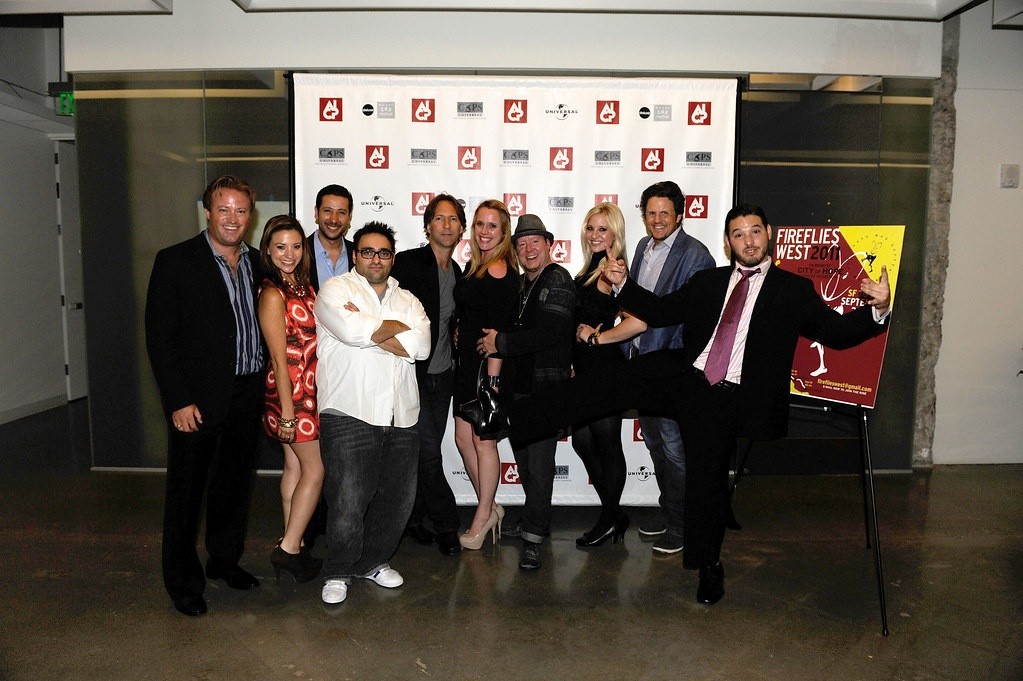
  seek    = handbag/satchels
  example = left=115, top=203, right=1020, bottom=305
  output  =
left=478, top=379, right=503, bottom=427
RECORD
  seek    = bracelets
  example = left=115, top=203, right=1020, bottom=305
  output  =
left=588, top=333, right=600, bottom=347
left=278, top=417, right=298, bottom=428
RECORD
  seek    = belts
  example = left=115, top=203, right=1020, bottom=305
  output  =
left=689, top=366, right=743, bottom=398
left=231, top=371, right=265, bottom=386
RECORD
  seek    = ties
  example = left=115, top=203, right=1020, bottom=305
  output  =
left=703, top=268, right=762, bottom=386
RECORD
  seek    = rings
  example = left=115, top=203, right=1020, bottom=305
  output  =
left=177, top=424, right=182, bottom=427
left=286, top=436, right=290, bottom=439
left=482, top=349, right=484, bottom=352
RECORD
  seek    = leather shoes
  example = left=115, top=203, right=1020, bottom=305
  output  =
left=697, top=559, right=724, bottom=605
left=406, top=523, right=436, bottom=545
left=205, top=562, right=260, bottom=590
left=496, top=522, right=523, bottom=537
left=173, top=586, right=207, bottom=617
left=435, top=528, right=462, bottom=556
left=724, top=505, right=742, bottom=532
left=519, top=541, right=541, bottom=567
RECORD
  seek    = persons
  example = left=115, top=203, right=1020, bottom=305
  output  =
left=257, top=215, right=325, bottom=583
left=452, top=201, right=521, bottom=549
left=391, top=194, right=466, bottom=556
left=454, top=214, right=587, bottom=570
left=304, top=184, right=355, bottom=549
left=601, top=205, right=892, bottom=605
left=143, top=174, right=272, bottom=616
left=313, top=221, right=432, bottom=603
left=570, top=203, right=647, bottom=546
left=620, top=181, right=717, bottom=554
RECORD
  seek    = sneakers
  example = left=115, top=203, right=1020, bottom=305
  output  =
left=321, top=579, right=348, bottom=603
left=365, top=564, right=404, bottom=588
left=652, top=534, right=685, bottom=553
left=637, top=522, right=668, bottom=535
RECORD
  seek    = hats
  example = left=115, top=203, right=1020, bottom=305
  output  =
left=510, top=213, right=554, bottom=247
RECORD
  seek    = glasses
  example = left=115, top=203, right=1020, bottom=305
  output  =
left=357, top=249, right=394, bottom=260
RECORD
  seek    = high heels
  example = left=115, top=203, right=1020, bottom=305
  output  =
left=459, top=504, right=505, bottom=550
left=270, top=538, right=325, bottom=582
left=576, top=510, right=630, bottom=547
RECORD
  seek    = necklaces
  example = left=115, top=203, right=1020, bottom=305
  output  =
left=283, top=271, right=305, bottom=296
left=519, top=264, right=549, bottom=319
